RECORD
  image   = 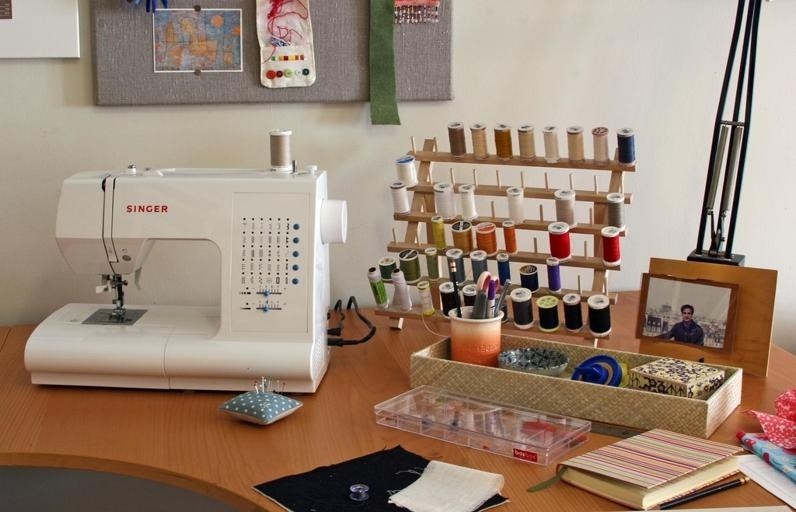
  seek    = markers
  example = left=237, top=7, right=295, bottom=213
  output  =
left=470, top=290, right=487, bottom=319
left=488, top=280, right=496, bottom=318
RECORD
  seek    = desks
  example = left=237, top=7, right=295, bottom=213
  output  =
left=1, top=290, right=796, bottom=512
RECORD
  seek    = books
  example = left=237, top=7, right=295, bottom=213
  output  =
left=556, top=426, right=745, bottom=511
left=737, top=430, right=796, bottom=483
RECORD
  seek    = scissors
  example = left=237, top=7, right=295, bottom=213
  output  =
left=476, top=271, right=500, bottom=293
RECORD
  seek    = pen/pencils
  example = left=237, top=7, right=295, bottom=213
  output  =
left=495, top=280, right=511, bottom=317
left=649, top=477, right=749, bottom=511
left=446, top=257, right=463, bottom=318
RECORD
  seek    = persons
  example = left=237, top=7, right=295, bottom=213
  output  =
left=653, top=304, right=705, bottom=347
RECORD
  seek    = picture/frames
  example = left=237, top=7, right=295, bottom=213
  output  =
left=637, top=272, right=737, bottom=350
